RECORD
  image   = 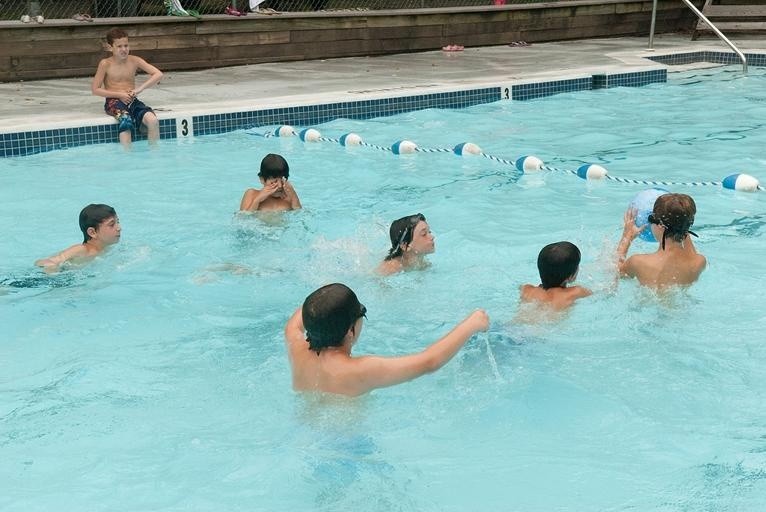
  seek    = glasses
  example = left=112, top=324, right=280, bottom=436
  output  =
left=400, top=212, right=426, bottom=241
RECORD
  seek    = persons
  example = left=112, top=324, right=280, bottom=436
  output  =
left=239, top=152, right=302, bottom=217
left=283, top=281, right=491, bottom=399
left=373, top=212, right=436, bottom=280
left=91, top=27, right=165, bottom=153
left=0, top=204, right=122, bottom=291
left=503, top=241, right=617, bottom=329
left=611, top=191, right=709, bottom=290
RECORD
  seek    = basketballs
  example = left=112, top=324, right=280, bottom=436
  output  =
left=630, top=188, right=672, bottom=242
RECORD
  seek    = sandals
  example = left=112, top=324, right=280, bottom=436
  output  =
left=510, top=40, right=532, bottom=47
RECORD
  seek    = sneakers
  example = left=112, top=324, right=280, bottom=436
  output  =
left=443, top=44, right=464, bottom=51
